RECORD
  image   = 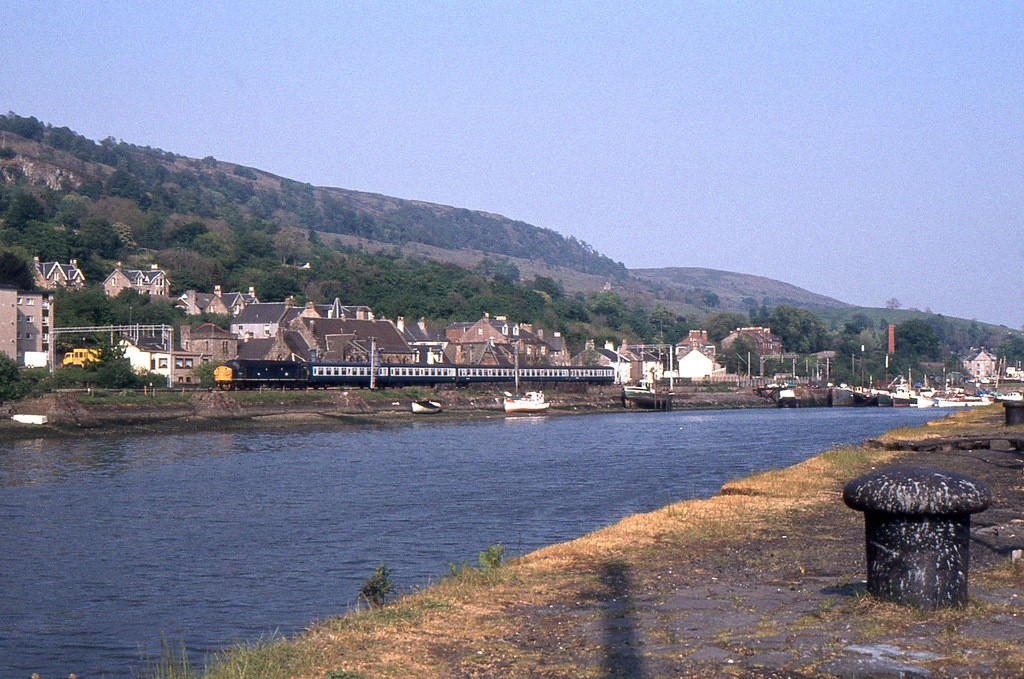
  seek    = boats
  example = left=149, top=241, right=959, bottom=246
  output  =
left=827, top=383, right=1024, bottom=408
left=411, top=400, right=442, bottom=414
left=623, top=385, right=673, bottom=411
left=503, top=391, right=550, bottom=414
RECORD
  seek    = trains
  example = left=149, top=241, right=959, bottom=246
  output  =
left=212, top=358, right=618, bottom=392
